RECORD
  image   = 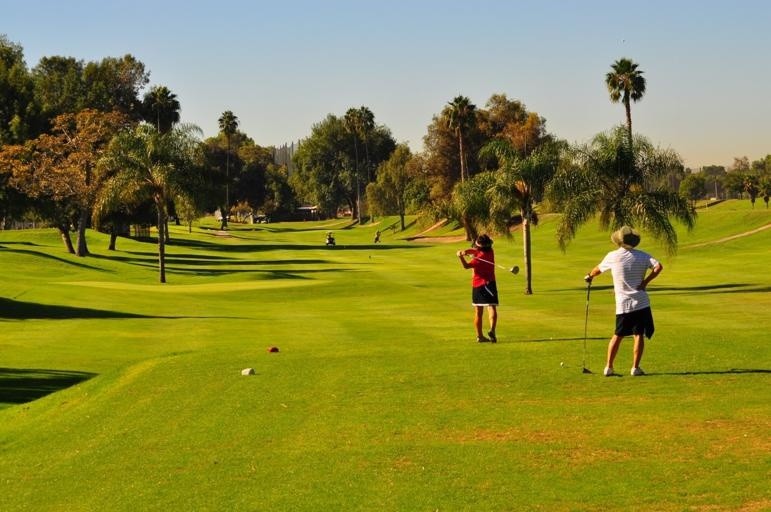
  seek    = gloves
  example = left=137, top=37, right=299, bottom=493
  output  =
left=584, top=272, right=594, bottom=282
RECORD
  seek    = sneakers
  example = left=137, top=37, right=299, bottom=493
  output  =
left=629, top=366, right=645, bottom=377
left=474, top=328, right=497, bottom=342
left=602, top=366, right=616, bottom=377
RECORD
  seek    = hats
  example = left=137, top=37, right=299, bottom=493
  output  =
left=611, top=224, right=641, bottom=249
left=472, top=234, right=494, bottom=250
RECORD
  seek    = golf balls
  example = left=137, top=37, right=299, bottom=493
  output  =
left=560, top=362, right=564, bottom=366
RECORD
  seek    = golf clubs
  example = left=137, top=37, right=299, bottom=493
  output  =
left=467, top=253, right=519, bottom=274
left=582, top=281, right=592, bottom=374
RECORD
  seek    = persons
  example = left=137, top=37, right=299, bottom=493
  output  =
left=457, top=234, right=498, bottom=344
left=584, top=225, right=663, bottom=376
left=375, top=231, right=381, bottom=243
